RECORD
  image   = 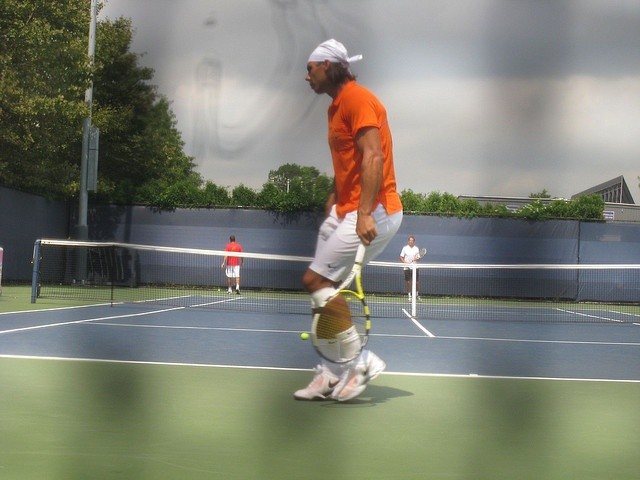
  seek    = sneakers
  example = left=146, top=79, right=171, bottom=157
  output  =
left=226, top=286, right=233, bottom=294
left=416, top=296, right=422, bottom=301
left=293, top=363, right=340, bottom=401
left=408, top=297, right=412, bottom=303
left=235, top=288, right=240, bottom=294
left=330, top=350, right=386, bottom=402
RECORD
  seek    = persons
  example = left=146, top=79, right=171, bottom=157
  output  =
left=293, top=38, right=404, bottom=402
left=400, top=235, right=422, bottom=302
left=221, top=234, right=243, bottom=294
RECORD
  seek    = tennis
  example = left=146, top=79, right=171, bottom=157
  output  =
left=301, top=332, right=309, bottom=341
left=218, top=289, right=221, bottom=292
